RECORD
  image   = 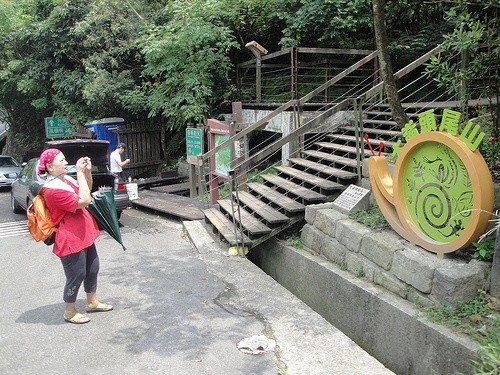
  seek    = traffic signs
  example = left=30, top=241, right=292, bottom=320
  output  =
left=45, top=117, right=74, bottom=138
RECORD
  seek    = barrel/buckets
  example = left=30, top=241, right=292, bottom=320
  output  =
left=126, top=183, right=138, bottom=200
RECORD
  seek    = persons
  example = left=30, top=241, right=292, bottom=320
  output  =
left=37, top=149, right=114, bottom=324
left=109, top=143, right=130, bottom=178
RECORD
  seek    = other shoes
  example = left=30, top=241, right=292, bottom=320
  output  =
left=85, top=302, right=113, bottom=312
left=64, top=311, right=90, bottom=324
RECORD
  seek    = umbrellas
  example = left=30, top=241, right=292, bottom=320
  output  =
left=85, top=185, right=127, bottom=252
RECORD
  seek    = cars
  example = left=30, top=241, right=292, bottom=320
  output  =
left=0, top=155, right=24, bottom=188
left=9, top=139, right=129, bottom=221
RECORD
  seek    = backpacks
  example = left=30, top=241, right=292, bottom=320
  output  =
left=26, top=183, right=68, bottom=246
